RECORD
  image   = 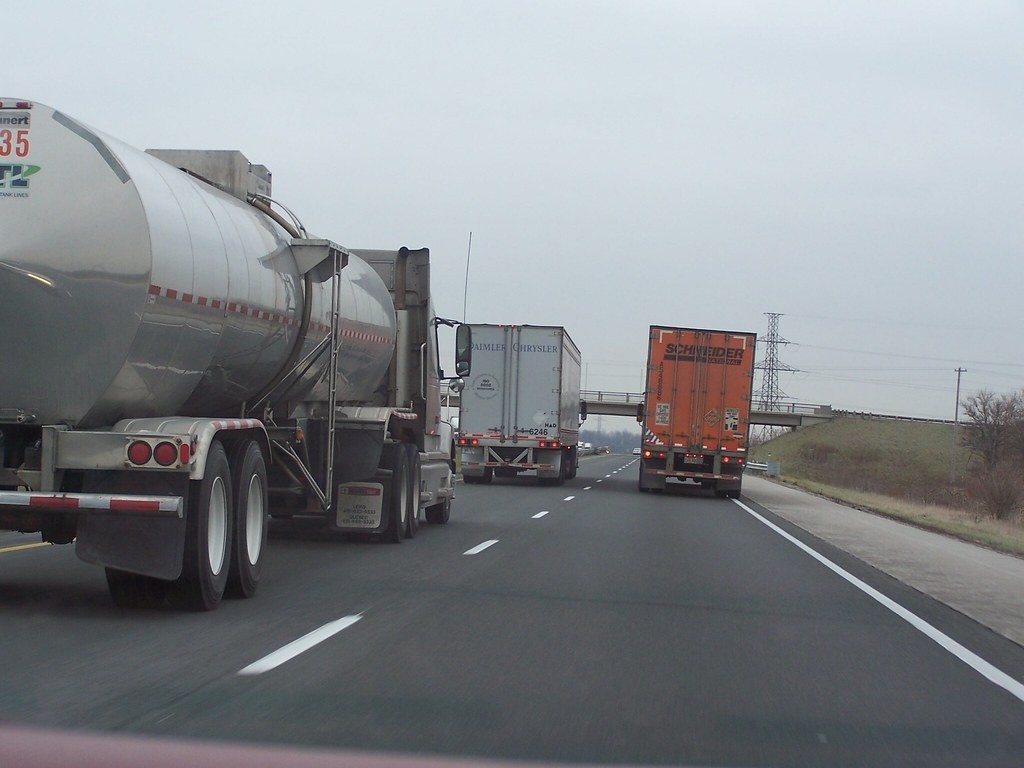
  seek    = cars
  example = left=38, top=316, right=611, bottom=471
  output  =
left=576, top=440, right=611, bottom=457
left=633, top=447, right=642, bottom=453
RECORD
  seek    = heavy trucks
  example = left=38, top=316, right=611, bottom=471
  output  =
left=457, top=323, right=588, bottom=486
left=636, top=325, right=758, bottom=499
left=1, top=95, right=471, bottom=614
